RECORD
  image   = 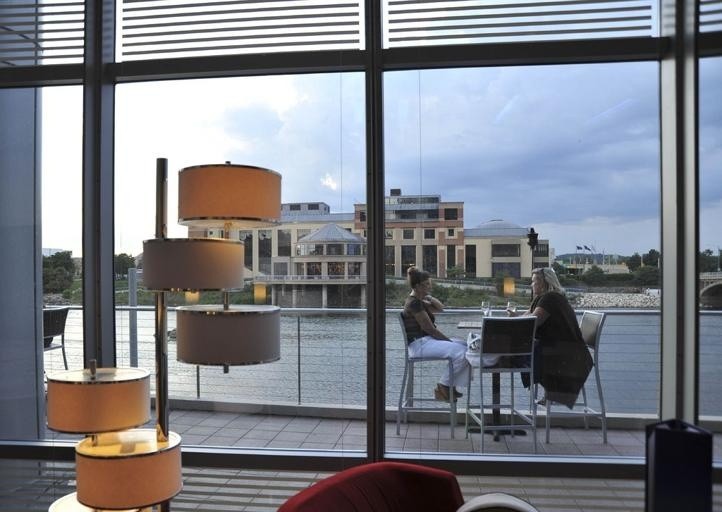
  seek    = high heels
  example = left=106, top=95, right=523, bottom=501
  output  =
left=434, top=383, right=464, bottom=402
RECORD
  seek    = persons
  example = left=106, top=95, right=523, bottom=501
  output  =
left=403, top=266, right=470, bottom=404
left=509, top=267, right=593, bottom=407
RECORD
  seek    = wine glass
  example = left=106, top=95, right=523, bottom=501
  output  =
left=482, top=301, right=490, bottom=317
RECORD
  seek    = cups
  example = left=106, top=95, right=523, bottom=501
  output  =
left=506, top=301, right=516, bottom=316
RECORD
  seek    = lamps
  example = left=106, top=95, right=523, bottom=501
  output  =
left=46, top=156, right=285, bottom=512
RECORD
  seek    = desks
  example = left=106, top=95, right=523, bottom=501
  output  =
left=456, top=320, right=530, bottom=441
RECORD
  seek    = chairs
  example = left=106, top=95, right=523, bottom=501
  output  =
left=396, top=309, right=459, bottom=440
left=532, top=309, right=609, bottom=446
left=464, top=315, right=539, bottom=454
left=43, top=307, right=72, bottom=370
left=276, top=462, right=465, bottom=512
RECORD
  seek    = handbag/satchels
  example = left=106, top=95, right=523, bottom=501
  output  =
left=467, top=332, right=481, bottom=353
